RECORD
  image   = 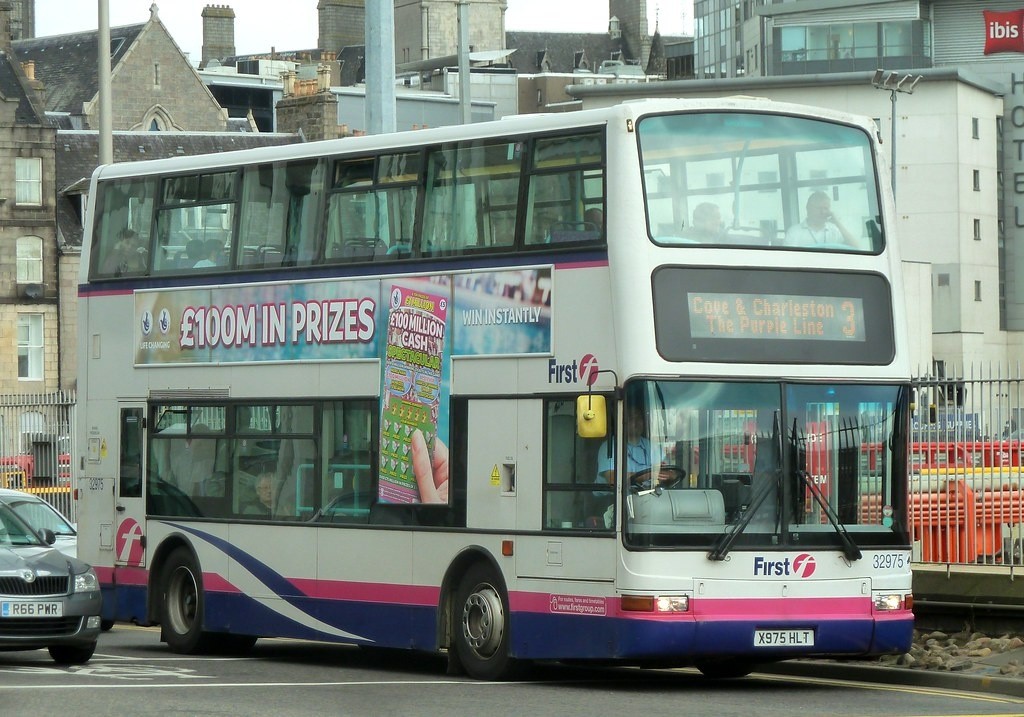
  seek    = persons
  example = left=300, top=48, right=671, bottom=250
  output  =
left=594, top=402, right=676, bottom=515
left=245, top=472, right=272, bottom=515
left=783, top=191, right=864, bottom=249
left=693, top=202, right=720, bottom=233
left=583, top=207, right=603, bottom=229
left=103, top=229, right=147, bottom=275
left=186, top=239, right=224, bottom=268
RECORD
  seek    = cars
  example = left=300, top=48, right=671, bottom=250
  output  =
left=1, top=499, right=103, bottom=665
left=1, top=487, right=78, bottom=559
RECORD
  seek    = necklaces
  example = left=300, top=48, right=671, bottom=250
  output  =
left=809, top=229, right=827, bottom=246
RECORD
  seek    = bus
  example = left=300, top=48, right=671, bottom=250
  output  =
left=70, top=99, right=916, bottom=682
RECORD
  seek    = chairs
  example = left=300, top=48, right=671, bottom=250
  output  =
left=103, top=222, right=790, bottom=279
